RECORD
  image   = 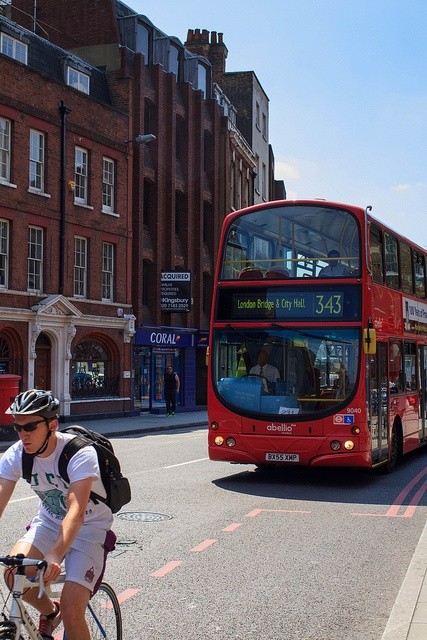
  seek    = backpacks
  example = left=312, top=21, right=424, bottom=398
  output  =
left=58, top=425, right=131, bottom=514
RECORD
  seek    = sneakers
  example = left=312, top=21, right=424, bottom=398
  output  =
left=172, top=411, right=175, bottom=415
left=166, top=413, right=171, bottom=417
left=39, top=602, right=63, bottom=636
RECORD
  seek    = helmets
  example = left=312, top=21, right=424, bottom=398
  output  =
left=5, top=390, right=61, bottom=419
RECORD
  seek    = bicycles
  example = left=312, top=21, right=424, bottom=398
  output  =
left=0, top=552, right=121, bottom=640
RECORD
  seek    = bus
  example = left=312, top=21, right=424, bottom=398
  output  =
left=206, top=199, right=426, bottom=474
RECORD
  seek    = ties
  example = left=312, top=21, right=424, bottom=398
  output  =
left=260, top=367, right=263, bottom=377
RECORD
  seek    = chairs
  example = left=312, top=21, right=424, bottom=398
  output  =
left=238, top=266, right=262, bottom=278
left=265, top=266, right=290, bottom=278
left=320, top=391, right=342, bottom=410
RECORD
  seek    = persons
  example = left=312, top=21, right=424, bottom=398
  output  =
left=249, top=350, right=280, bottom=383
left=159, top=366, right=180, bottom=417
left=1, top=389, right=113, bottom=640
left=318, top=250, right=349, bottom=277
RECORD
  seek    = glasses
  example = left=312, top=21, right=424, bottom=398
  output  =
left=168, top=368, right=172, bottom=370
left=12, top=421, right=49, bottom=432
